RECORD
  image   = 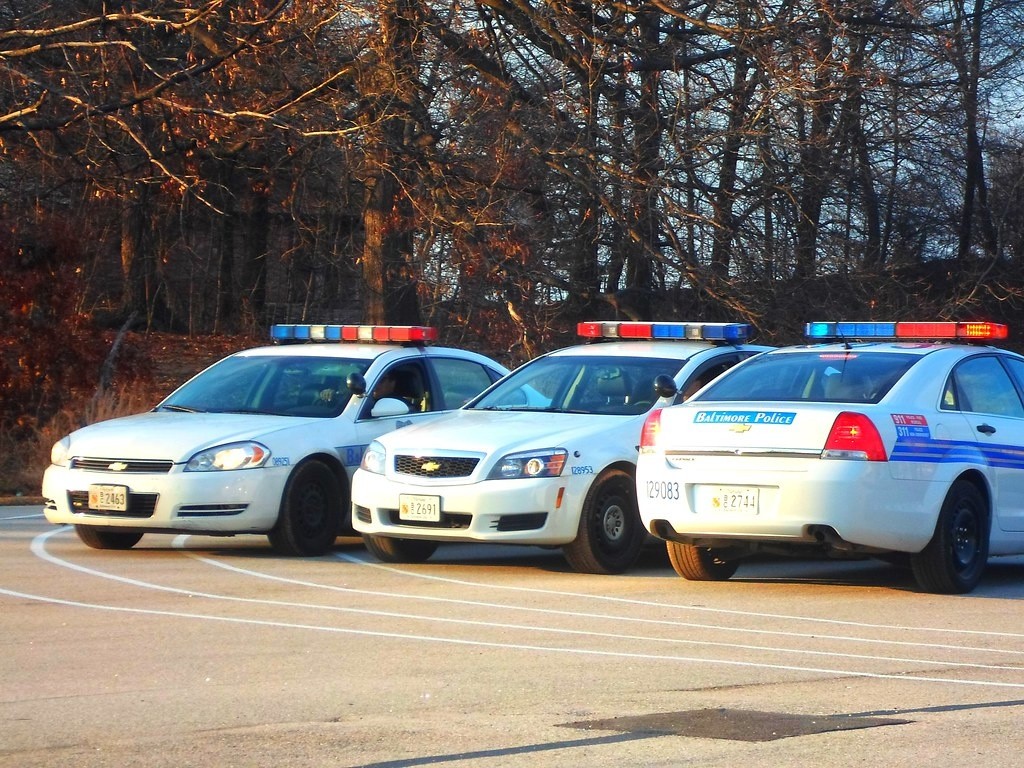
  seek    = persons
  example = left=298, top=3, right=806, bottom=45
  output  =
left=682, top=373, right=702, bottom=403
left=318, top=368, right=416, bottom=420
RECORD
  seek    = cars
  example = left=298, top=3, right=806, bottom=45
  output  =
left=40, top=324, right=555, bottom=560
left=634, top=320, right=1024, bottom=595
left=351, top=321, right=783, bottom=574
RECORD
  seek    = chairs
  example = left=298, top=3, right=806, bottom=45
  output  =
left=298, top=383, right=330, bottom=406
left=824, top=373, right=863, bottom=399
left=596, top=371, right=632, bottom=405
